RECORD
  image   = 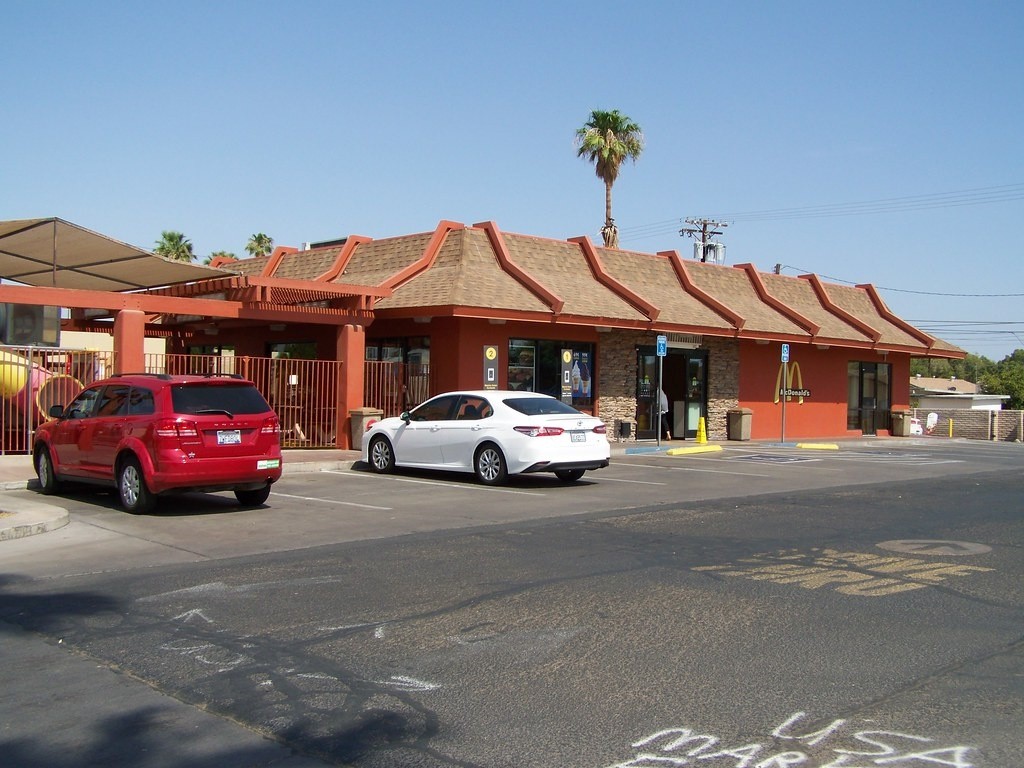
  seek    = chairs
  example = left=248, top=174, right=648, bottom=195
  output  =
left=457, top=405, right=483, bottom=420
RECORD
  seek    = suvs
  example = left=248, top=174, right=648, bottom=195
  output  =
left=32, top=373, right=284, bottom=515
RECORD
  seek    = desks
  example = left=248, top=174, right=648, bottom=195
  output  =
left=311, top=406, right=336, bottom=442
left=275, top=404, right=305, bottom=441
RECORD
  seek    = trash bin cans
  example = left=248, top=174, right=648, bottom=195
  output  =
left=891, top=410, right=913, bottom=437
left=348, top=407, right=383, bottom=451
left=728, top=408, right=753, bottom=440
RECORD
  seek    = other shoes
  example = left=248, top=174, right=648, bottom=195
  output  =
left=666, top=436, right=671, bottom=441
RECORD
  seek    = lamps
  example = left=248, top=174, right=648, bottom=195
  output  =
left=414, top=317, right=431, bottom=323
left=593, top=327, right=613, bottom=333
left=816, top=345, right=830, bottom=350
left=876, top=350, right=890, bottom=355
left=755, top=339, right=770, bottom=345
left=204, top=329, right=220, bottom=335
left=269, top=324, right=287, bottom=331
left=488, top=319, right=506, bottom=324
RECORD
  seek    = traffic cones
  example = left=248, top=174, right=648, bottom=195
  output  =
left=695, top=417, right=708, bottom=445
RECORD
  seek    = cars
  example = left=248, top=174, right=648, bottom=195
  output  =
left=360, top=389, right=610, bottom=487
left=910, top=418, right=924, bottom=436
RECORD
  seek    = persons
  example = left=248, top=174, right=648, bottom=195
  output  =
left=656, top=381, right=671, bottom=440
